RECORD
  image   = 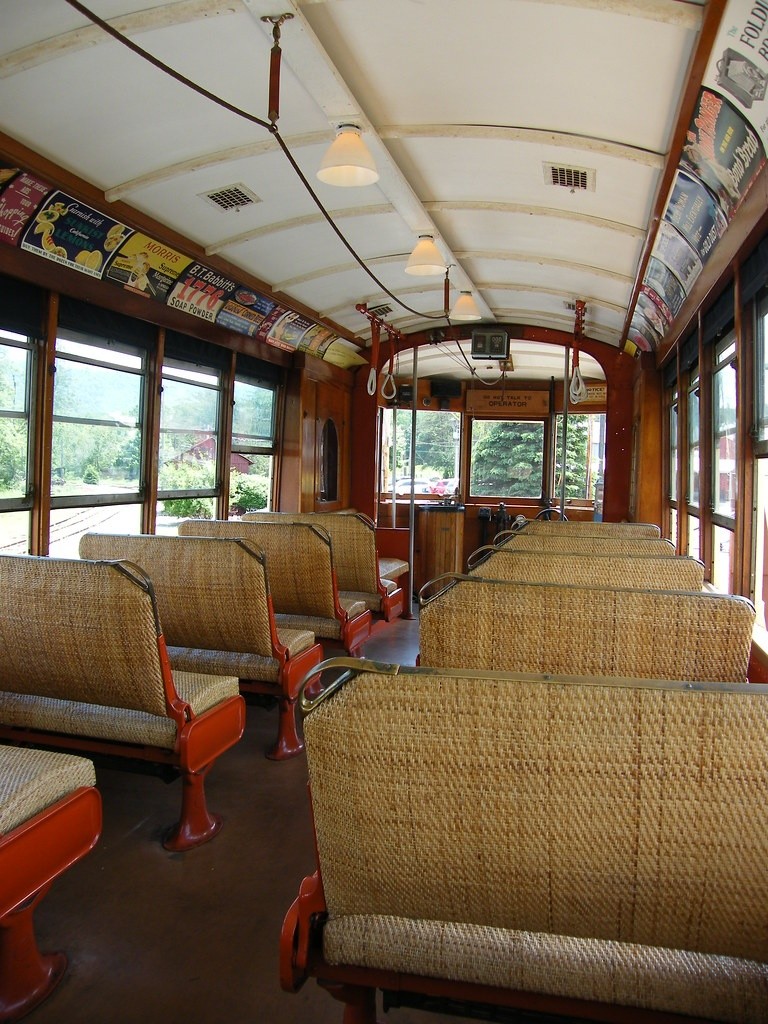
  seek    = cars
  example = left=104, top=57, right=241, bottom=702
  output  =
left=388, top=476, right=457, bottom=494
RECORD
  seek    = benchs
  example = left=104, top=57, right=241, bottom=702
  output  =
left=276, top=519, right=768, bottom=1024
left=0, top=496, right=411, bottom=1024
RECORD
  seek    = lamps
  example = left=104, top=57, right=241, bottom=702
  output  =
left=449, top=288, right=482, bottom=321
left=404, top=229, right=447, bottom=277
left=315, top=115, right=378, bottom=187
